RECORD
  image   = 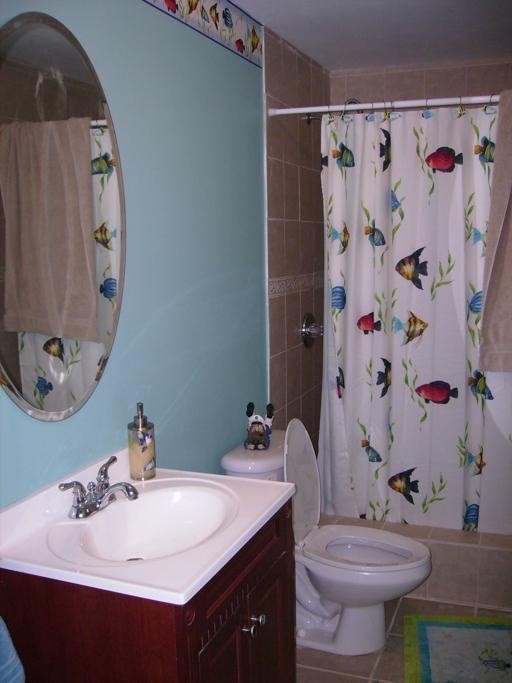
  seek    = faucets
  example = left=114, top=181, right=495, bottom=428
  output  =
left=58, top=454, right=139, bottom=519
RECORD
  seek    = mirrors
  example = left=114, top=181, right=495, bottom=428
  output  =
left=0, top=11, right=125, bottom=422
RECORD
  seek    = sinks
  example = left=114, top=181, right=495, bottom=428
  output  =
left=40, top=471, right=245, bottom=573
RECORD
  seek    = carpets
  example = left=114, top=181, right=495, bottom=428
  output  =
left=402, top=613, right=512, bottom=683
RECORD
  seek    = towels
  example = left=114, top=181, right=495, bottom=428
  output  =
left=0, top=117, right=102, bottom=345
left=478, top=89, right=512, bottom=375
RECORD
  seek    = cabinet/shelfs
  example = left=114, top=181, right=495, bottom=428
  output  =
left=0, top=497, right=296, bottom=683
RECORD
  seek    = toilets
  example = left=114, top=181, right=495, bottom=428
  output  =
left=222, top=418, right=432, bottom=655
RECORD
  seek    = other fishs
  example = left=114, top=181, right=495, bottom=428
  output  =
left=321, top=102, right=496, bottom=534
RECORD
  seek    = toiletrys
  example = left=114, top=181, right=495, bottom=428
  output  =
left=128, top=400, right=157, bottom=480
